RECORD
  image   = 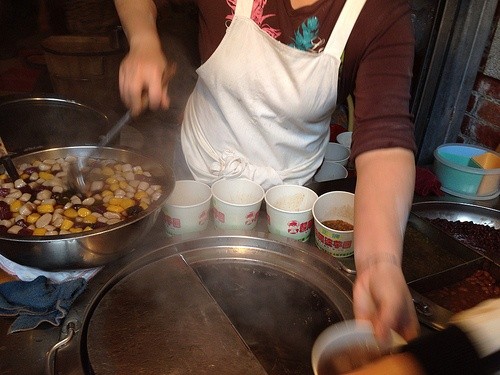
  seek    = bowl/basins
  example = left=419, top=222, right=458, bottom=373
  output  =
left=211, top=176, right=265, bottom=235
left=0, top=145, right=175, bottom=273
left=0, top=93, right=120, bottom=153
left=336, top=132, right=354, bottom=146
left=312, top=191, right=355, bottom=258
left=314, top=162, right=349, bottom=183
left=325, top=142, right=350, bottom=167
left=311, top=319, right=408, bottom=375
left=265, top=184, right=318, bottom=243
left=164, top=180, right=213, bottom=242
left=432, top=143, right=500, bottom=197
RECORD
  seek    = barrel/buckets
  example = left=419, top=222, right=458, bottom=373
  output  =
left=40, top=29, right=129, bottom=108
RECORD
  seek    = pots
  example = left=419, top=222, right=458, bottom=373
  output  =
left=47, top=230, right=355, bottom=375
left=402, top=201, right=500, bottom=333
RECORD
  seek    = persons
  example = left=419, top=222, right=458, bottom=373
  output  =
left=113, top=0, right=421, bottom=349
